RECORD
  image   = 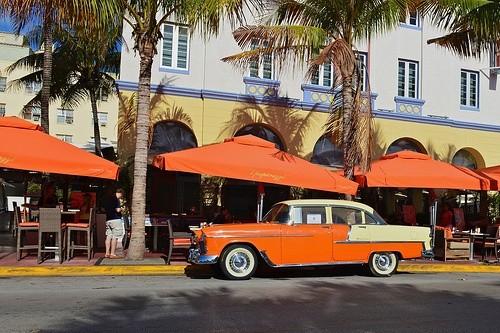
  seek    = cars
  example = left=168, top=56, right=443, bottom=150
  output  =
left=187, top=199, right=433, bottom=280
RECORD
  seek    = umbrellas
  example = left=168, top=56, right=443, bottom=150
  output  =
left=0, top=115, right=121, bottom=182
left=151, top=133, right=500, bottom=224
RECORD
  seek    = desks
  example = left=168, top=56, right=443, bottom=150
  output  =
left=30, top=210, right=77, bottom=261
left=454, top=232, right=490, bottom=261
left=152, top=224, right=172, bottom=252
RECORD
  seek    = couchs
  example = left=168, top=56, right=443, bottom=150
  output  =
left=432, top=226, right=471, bottom=263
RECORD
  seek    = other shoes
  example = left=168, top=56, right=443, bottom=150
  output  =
left=110, top=254, right=121, bottom=258
left=105, top=255, right=111, bottom=258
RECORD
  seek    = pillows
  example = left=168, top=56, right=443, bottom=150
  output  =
left=435, top=226, right=453, bottom=239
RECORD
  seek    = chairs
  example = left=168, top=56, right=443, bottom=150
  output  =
left=473, top=225, right=499, bottom=260
left=122, top=213, right=195, bottom=265
left=12, top=201, right=95, bottom=264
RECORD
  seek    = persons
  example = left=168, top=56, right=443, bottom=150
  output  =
left=77, top=193, right=93, bottom=253
left=105, top=188, right=126, bottom=260
left=439, top=202, right=453, bottom=229
left=22, top=183, right=58, bottom=218
